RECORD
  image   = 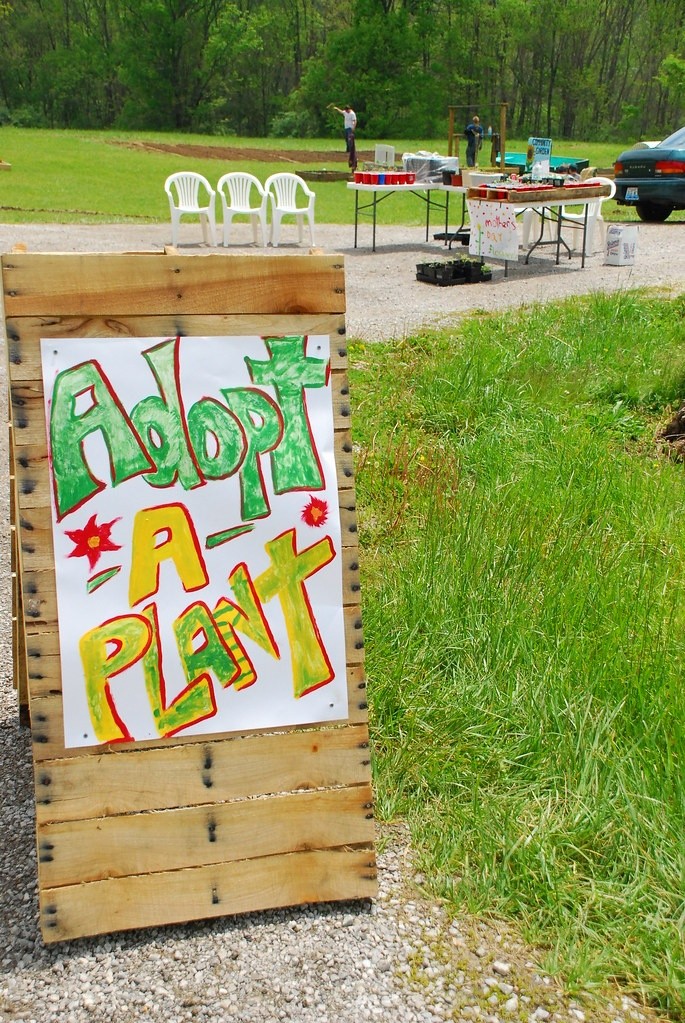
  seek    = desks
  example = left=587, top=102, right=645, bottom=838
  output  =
left=439, top=184, right=468, bottom=250
left=468, top=197, right=599, bottom=277
left=347, top=182, right=449, bottom=251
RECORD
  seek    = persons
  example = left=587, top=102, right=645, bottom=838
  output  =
left=334, top=105, right=357, bottom=152
left=464, top=116, right=483, bottom=167
left=559, top=164, right=581, bottom=179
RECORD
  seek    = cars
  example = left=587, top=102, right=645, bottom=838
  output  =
left=611, top=125, right=685, bottom=225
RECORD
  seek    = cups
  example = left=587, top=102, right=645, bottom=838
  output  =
left=442, top=170, right=455, bottom=185
left=451, top=174, right=462, bottom=186
left=354, top=171, right=415, bottom=184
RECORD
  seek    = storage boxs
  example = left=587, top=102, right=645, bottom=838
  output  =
left=407, top=157, right=459, bottom=182
left=605, top=224, right=640, bottom=265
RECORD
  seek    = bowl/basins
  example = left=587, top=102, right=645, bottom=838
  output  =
left=469, top=173, right=504, bottom=186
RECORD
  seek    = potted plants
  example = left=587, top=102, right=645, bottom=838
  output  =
left=415, top=252, right=491, bottom=280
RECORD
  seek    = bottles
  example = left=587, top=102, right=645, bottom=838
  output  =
left=531, top=161, right=543, bottom=180
left=488, top=126, right=493, bottom=137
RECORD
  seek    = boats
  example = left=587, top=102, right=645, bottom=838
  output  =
left=494, top=151, right=591, bottom=176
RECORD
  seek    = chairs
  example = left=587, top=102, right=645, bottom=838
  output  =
left=265, top=172, right=315, bottom=248
left=217, top=172, right=268, bottom=248
left=556, top=177, right=614, bottom=256
left=165, top=172, right=216, bottom=248
left=515, top=206, right=552, bottom=250
